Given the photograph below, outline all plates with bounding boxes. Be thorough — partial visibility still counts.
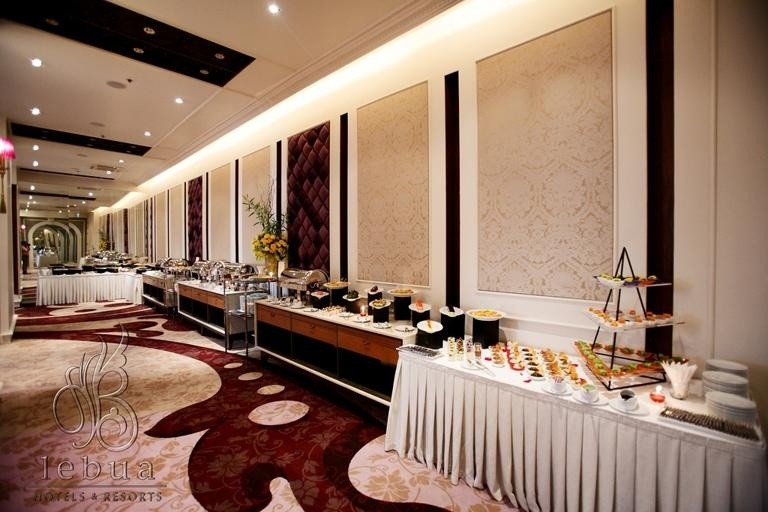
[271,299,317,313]
[238,293,267,314]
[489,339,648,416]
[144,271,176,281]
[183,278,232,291]
[703,391,757,424]
[311,280,506,337]
[703,359,748,374]
[700,371,750,396]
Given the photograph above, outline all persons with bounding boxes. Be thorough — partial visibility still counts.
[21,240,31,275]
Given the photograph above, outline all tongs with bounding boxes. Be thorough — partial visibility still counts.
[466,357,496,378]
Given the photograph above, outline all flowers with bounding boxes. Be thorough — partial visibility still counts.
[241,192,288,261]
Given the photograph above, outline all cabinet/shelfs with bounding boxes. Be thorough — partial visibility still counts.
[141,274,416,408]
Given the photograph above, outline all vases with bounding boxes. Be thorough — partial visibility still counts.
[265,256,278,278]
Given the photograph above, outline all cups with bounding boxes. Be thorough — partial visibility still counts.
[579,383,598,402]
[549,374,567,392]
[616,389,638,411]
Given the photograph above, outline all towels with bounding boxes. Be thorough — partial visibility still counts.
[660,359,698,396]
